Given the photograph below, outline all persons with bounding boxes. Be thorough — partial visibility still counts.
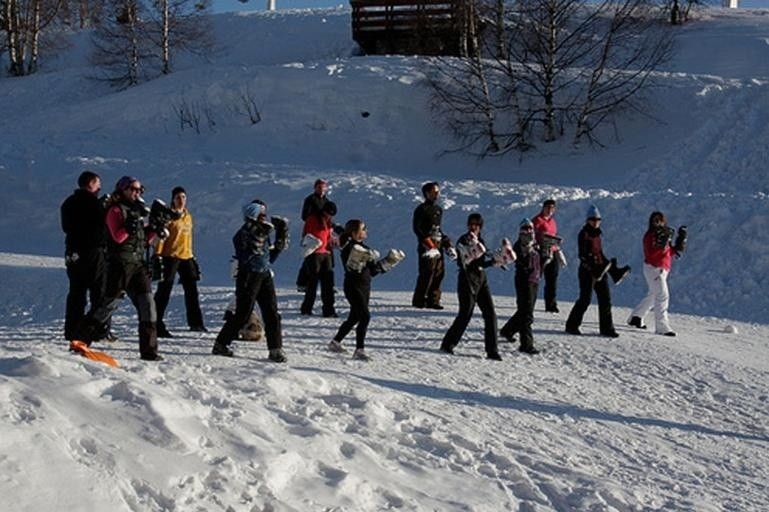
[297,179,342,292]
[627,211,687,337]
[532,199,567,314]
[60,171,117,341]
[301,202,342,318]
[565,205,632,338]
[225,200,272,341]
[413,182,458,309]
[441,213,517,360]
[71,171,166,362]
[328,220,405,362]
[152,187,208,337]
[500,217,542,353]
[212,200,290,363]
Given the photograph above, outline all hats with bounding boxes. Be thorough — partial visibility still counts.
[315,178,328,188]
[519,217,536,228]
[323,202,337,216]
[586,204,602,220]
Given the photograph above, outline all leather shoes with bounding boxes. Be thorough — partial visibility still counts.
[105,330,118,343]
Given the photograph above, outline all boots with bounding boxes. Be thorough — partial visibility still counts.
[213,341,234,356]
[301,234,322,257]
[421,238,441,260]
[126,195,150,238]
[608,258,632,287]
[329,233,351,250]
[519,231,536,258]
[444,240,457,263]
[492,238,518,267]
[378,248,406,273]
[150,256,165,283]
[677,225,689,252]
[335,224,344,234]
[654,224,674,247]
[457,231,487,265]
[541,232,563,256]
[585,258,612,282]
[180,261,201,284]
[268,349,286,363]
[245,214,274,247]
[346,245,380,273]
[270,215,290,251]
[148,198,182,240]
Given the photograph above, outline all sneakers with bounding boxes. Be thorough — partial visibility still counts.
[600,329,621,338]
[190,324,209,331]
[302,311,313,316]
[627,316,647,330]
[486,353,503,361]
[352,349,372,363]
[545,306,558,311]
[499,330,517,343]
[71,341,90,353]
[141,354,163,362]
[441,345,454,355]
[157,331,171,338]
[328,340,346,353]
[428,303,443,309]
[565,325,582,335]
[412,302,426,308]
[324,312,338,318]
[519,345,540,355]
[297,285,310,292]
[332,286,340,293]
[657,330,675,334]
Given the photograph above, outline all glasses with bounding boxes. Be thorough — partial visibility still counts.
[362,224,368,231]
[590,218,602,222]
[259,213,270,220]
[545,204,557,209]
[128,184,146,194]
